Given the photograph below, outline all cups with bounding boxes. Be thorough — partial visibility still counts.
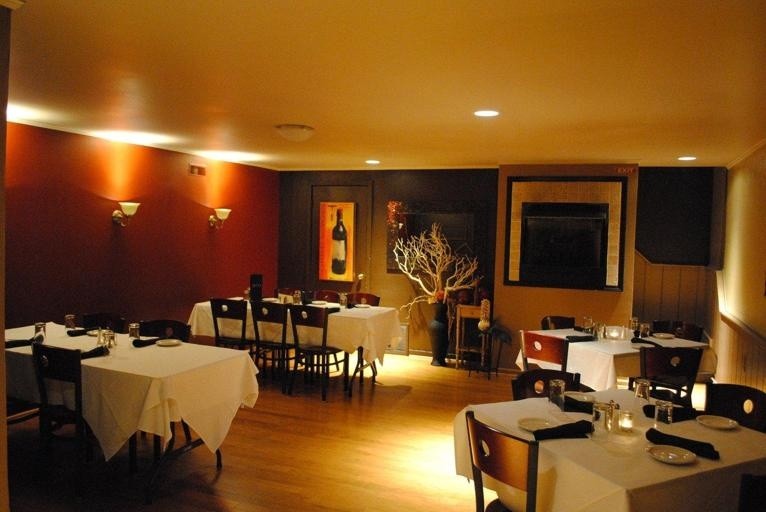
[634,379,650,408]
[654,400,673,431]
[596,322,606,340]
[65,315,76,333]
[583,317,594,333]
[628,316,639,330]
[639,324,650,339]
[35,322,46,342]
[128,323,141,344]
[591,402,614,442]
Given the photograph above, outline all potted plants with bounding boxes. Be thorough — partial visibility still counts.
[392,223,482,366]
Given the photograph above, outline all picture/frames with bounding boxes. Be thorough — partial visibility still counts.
[319,202,355,282]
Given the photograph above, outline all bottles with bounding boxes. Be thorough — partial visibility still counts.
[332,208,347,274]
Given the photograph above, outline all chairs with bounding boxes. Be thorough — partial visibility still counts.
[346,292,380,307]
[464,410,539,512]
[706,381,766,433]
[32,340,107,468]
[539,315,576,331]
[276,286,311,304]
[209,297,254,362]
[511,369,581,401]
[250,300,289,392]
[519,328,569,372]
[652,319,671,335]
[313,290,340,304]
[672,320,704,342]
[289,304,349,402]
[83,314,126,334]
[629,346,703,409]
[131,319,193,461]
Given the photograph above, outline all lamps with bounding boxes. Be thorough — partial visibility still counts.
[209,208,232,229]
[113,202,140,227]
[276,124,316,144]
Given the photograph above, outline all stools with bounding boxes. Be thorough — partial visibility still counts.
[455,302,491,369]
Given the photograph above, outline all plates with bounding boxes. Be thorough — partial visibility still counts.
[696,415,739,431]
[654,333,676,339]
[355,304,370,308]
[87,329,112,336]
[312,301,326,305]
[156,339,182,346]
[517,418,555,432]
[630,342,655,349]
[647,444,697,465]
[262,298,278,302]
[564,392,596,403]
